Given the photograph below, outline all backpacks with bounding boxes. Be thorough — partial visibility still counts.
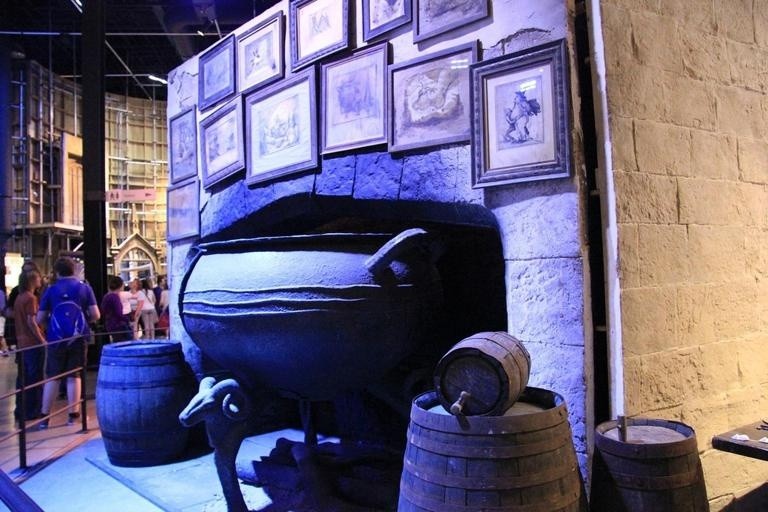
[47,281,90,360]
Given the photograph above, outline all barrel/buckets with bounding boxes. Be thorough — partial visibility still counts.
[94,337,195,468]
[394,385,589,512]
[433,330,532,419]
[587,411,711,512]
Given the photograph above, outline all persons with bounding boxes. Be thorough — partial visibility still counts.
[12,270,49,421]
[37,271,54,296]
[100,276,168,343]
[35,256,101,430]
[3,261,47,320]
[0,281,17,357]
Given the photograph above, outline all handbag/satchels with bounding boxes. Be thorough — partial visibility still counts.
[153,311,159,324]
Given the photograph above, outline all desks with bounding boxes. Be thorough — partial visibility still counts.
[710,417,768,463]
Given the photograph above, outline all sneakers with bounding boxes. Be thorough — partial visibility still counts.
[67,416,89,426]
[39,420,48,428]
[0,350,8,356]
